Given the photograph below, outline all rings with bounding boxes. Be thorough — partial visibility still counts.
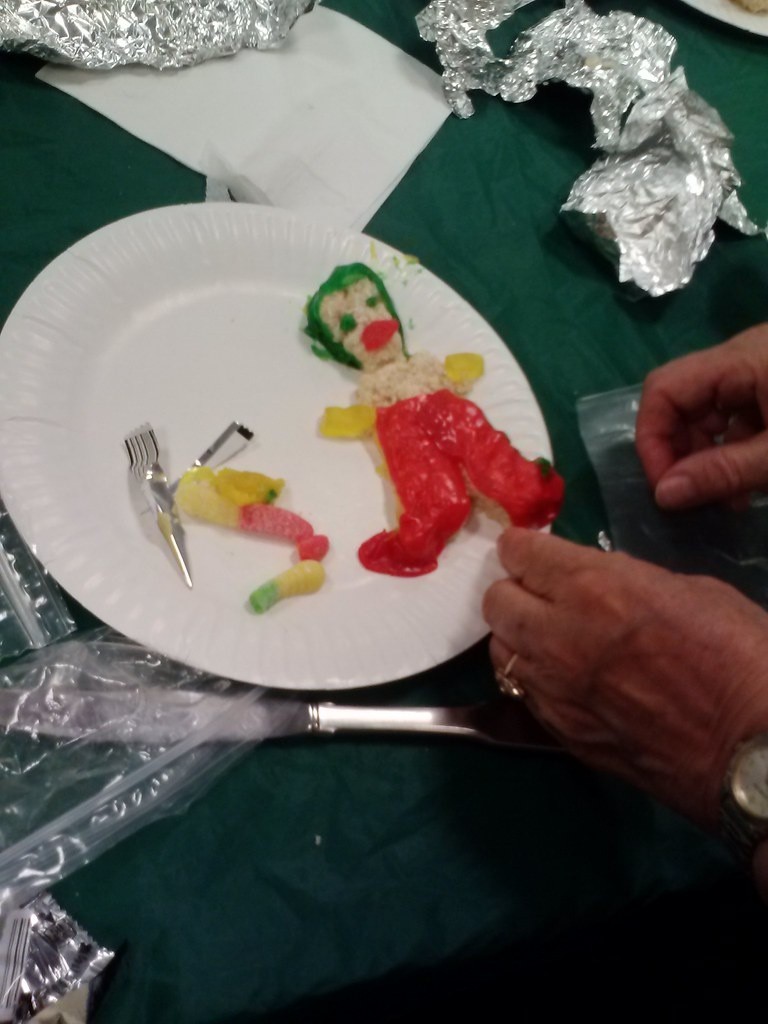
[495,653,524,698]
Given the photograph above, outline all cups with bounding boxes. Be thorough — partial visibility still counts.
[578,384,703,573]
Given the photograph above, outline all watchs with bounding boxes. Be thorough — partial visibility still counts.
[717,731,768,874]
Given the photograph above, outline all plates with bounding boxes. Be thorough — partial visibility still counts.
[681,0,768,48]
[1,201,559,692]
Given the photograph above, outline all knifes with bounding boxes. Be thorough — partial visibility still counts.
[0,683,553,753]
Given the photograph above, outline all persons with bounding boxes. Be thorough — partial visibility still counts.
[483,324,768,910]
